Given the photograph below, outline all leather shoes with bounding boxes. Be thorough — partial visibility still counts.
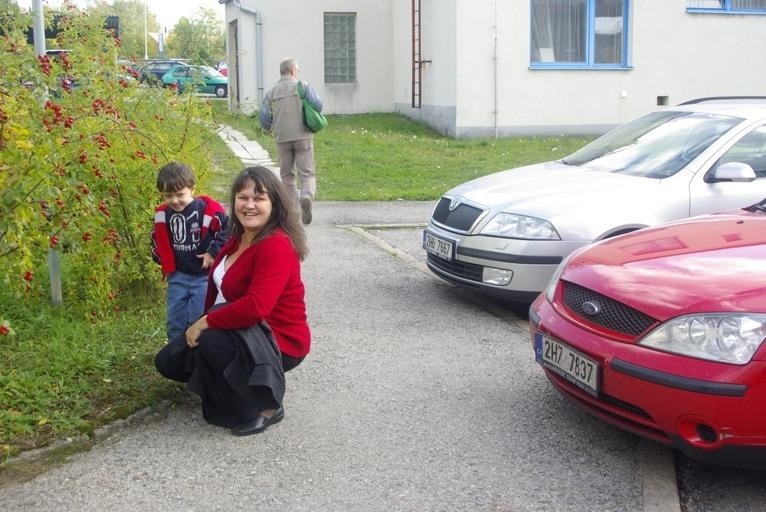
[231,405,285,436]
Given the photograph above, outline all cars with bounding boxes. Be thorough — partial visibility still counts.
[528,195,764,475]
[421,89,766,303]
[18,46,227,101]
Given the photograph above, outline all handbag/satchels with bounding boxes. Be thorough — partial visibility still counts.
[297,80,328,132]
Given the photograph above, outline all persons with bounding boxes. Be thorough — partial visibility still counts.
[151,162,228,347]
[154,167,310,435]
[260,58,323,225]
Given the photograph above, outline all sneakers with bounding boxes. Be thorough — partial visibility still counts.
[300,195,313,225]
